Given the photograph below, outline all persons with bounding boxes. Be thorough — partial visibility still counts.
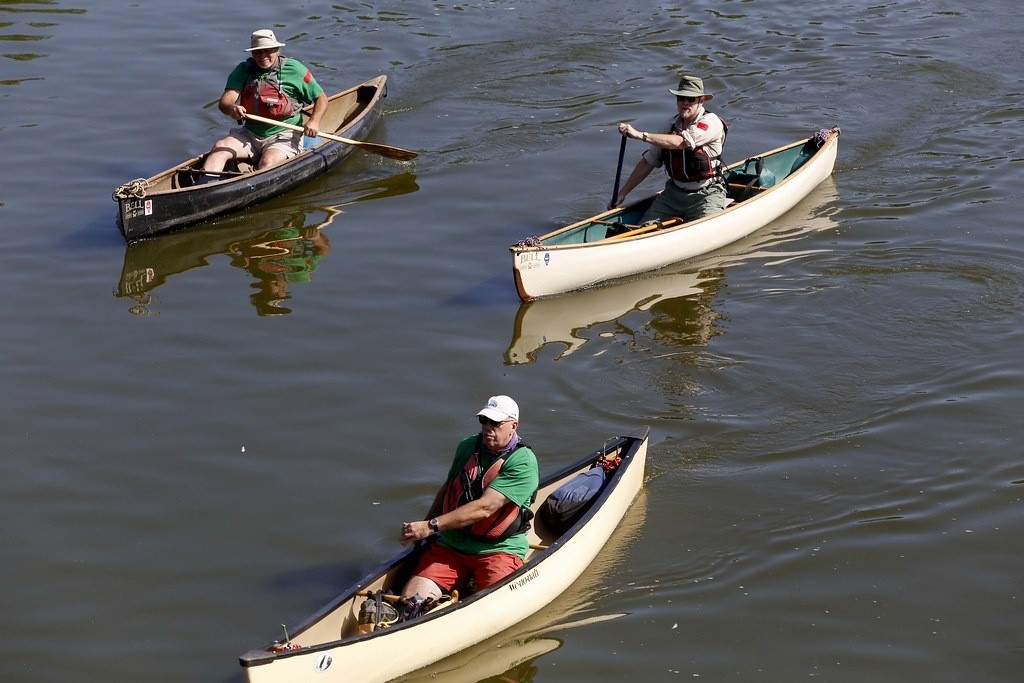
[197,28,329,186]
[606,75,728,234]
[399,396,540,607]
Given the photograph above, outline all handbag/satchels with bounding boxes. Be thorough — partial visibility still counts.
[726,157,775,202]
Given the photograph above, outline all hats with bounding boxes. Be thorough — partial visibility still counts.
[476,395,519,422]
[244,29,286,52]
[668,76,713,101]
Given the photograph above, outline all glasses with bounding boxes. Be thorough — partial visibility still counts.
[675,95,703,102]
[479,415,503,427]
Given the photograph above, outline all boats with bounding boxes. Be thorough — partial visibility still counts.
[508,123,840,299]
[118,127,382,299]
[237,422,650,683]
[111,72,388,241]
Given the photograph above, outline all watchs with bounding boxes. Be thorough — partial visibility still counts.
[429,518,439,533]
[643,132,649,142]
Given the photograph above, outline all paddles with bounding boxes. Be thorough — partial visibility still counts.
[243,113,419,161]
[610,134,627,209]
